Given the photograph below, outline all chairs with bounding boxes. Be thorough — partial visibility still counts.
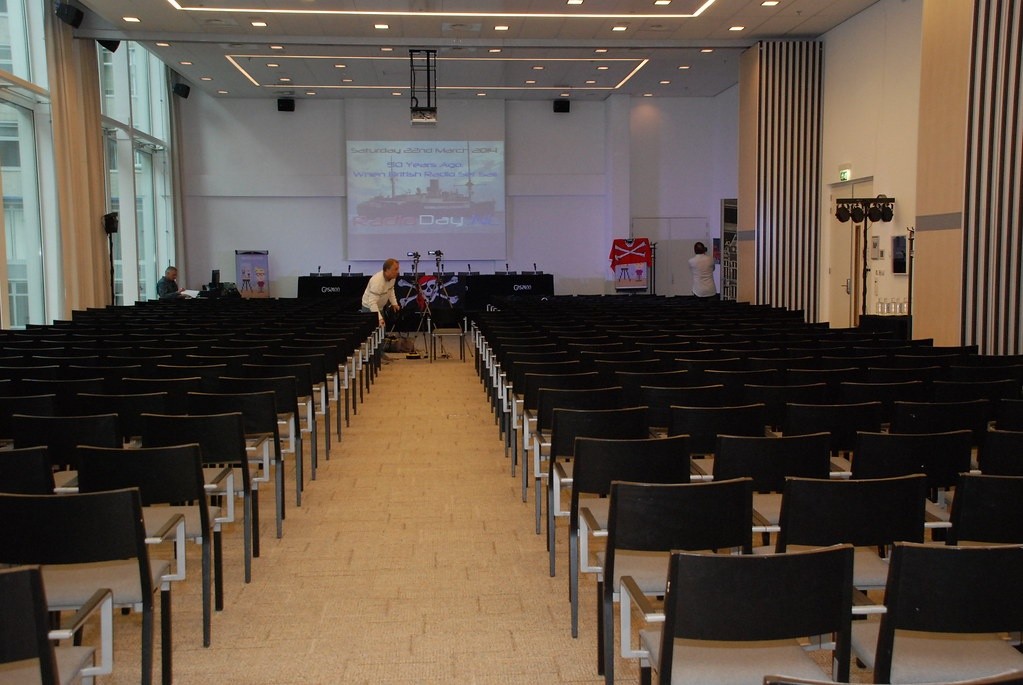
[0,294,1023,685]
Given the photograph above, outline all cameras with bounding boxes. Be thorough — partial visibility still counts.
[407,252,420,257]
[428,250,443,256]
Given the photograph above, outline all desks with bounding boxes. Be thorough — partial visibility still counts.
[297,277,555,325]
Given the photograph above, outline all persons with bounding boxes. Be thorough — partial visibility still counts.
[362,259,399,363]
[688,242,717,300]
[157,266,185,299]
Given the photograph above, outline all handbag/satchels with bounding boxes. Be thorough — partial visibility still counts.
[382,335,416,353]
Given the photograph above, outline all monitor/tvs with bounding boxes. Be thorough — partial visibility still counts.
[211,269,220,288]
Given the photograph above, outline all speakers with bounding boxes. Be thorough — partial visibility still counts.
[278,98,295,111]
[553,101,570,112]
[174,83,197,104]
[56,4,84,29]
[104,212,118,233]
[97,40,120,53]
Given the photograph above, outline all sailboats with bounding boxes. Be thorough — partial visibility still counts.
[357,141,494,222]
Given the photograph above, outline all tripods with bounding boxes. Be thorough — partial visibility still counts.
[382,256,474,358]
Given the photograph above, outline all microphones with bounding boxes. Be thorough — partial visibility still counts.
[318,266,322,270]
[412,264,414,272]
[506,264,508,269]
[467,264,470,270]
[442,264,444,268]
[534,263,536,270]
[348,265,351,270]
